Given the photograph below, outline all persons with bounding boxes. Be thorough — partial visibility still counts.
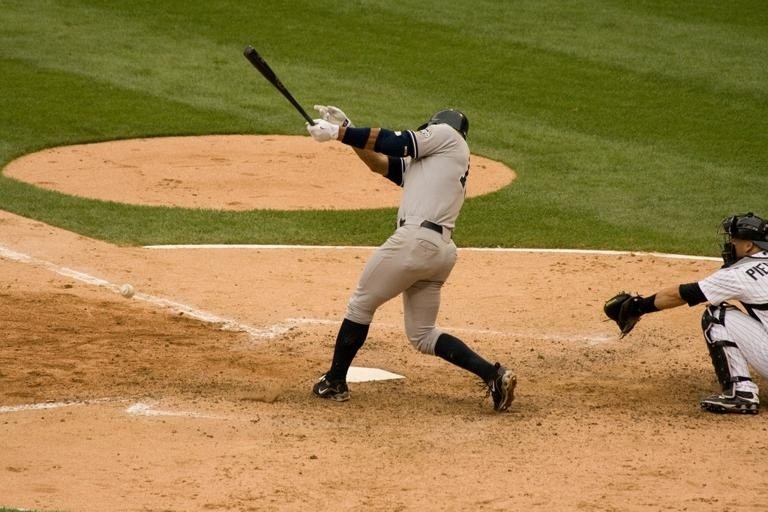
[603,210,767,417]
[306,103,520,414]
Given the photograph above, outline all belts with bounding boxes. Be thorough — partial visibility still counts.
[396,218,452,237]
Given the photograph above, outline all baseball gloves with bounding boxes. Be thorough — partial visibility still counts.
[604,292,644,338]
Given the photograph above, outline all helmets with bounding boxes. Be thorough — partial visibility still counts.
[416,107,468,139]
[715,213,768,268]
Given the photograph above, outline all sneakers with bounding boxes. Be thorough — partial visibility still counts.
[700,395,759,414]
[487,362,516,411]
[314,379,350,401]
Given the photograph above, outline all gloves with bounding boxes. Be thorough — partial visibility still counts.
[313,103,355,128]
[305,120,339,142]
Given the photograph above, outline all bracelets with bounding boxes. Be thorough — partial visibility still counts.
[342,124,371,151]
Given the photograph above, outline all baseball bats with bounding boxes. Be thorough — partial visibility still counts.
[244,45,314,125]
